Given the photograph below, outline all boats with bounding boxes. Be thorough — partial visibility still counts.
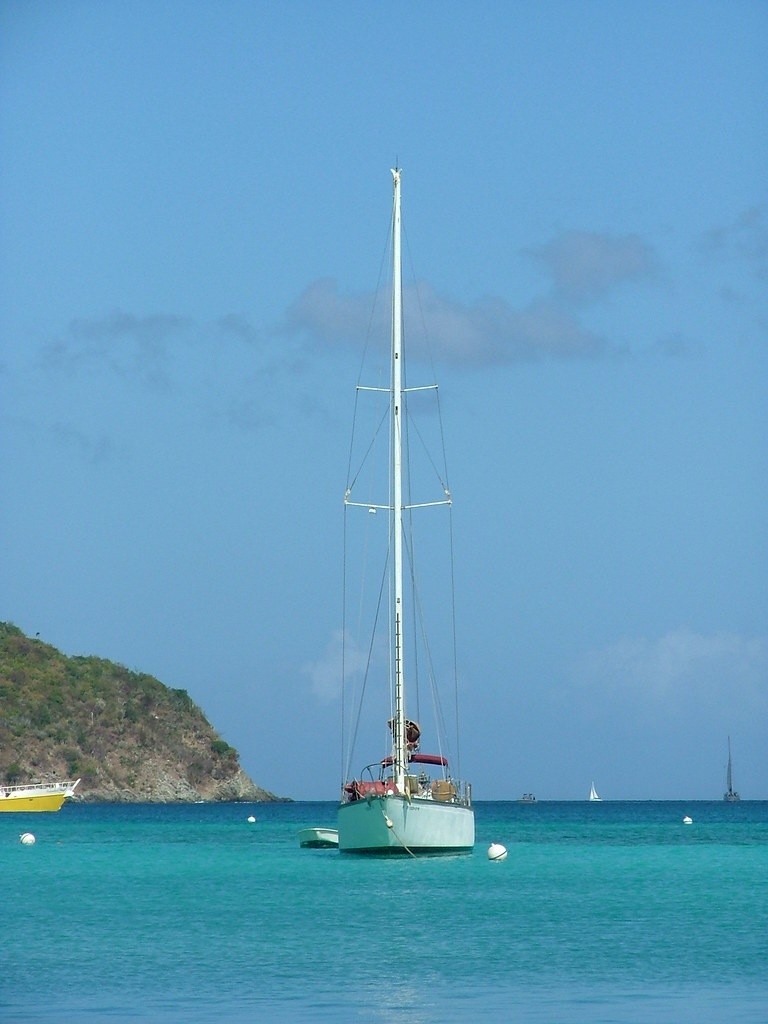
[517,791,538,804]
[0,778,82,813]
[297,828,339,845]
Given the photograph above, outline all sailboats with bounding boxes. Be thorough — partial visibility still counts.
[588,781,603,801]
[723,735,740,802]
[334,161,476,851]
